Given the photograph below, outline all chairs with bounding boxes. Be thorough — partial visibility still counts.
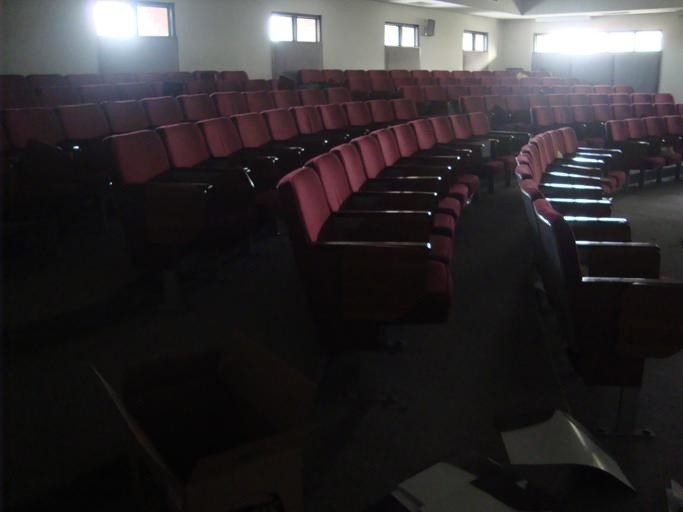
[0,68,683,368]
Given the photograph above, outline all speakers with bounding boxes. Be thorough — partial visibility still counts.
[425,19,435,36]
